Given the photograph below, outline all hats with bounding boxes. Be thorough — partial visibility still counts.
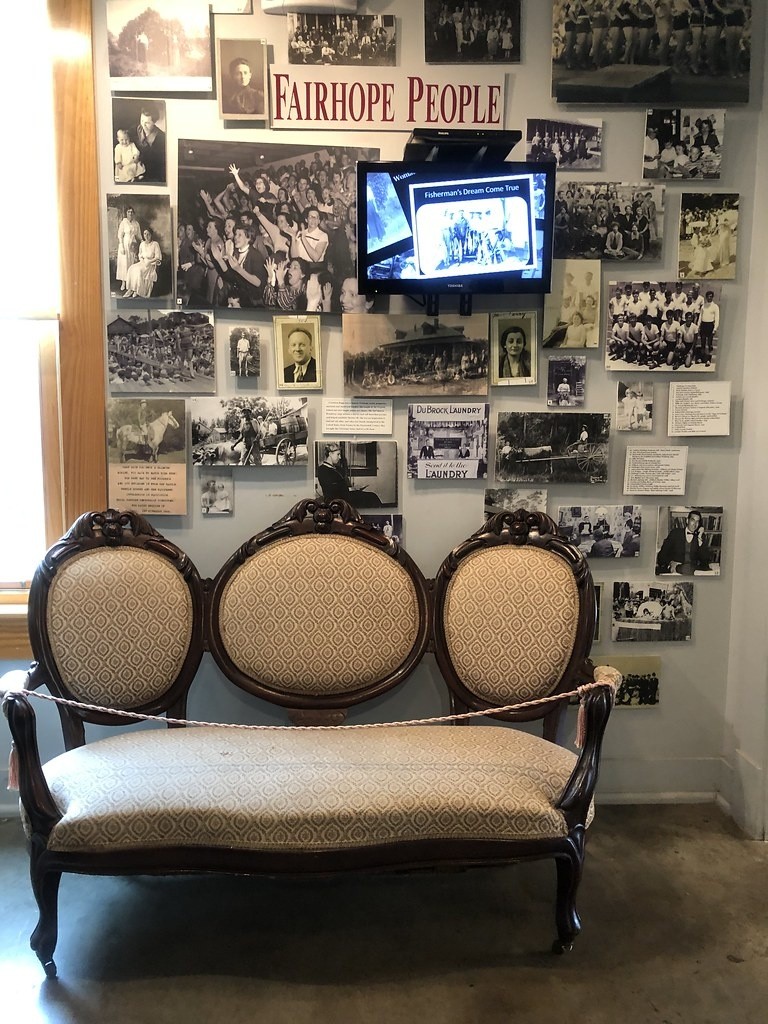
[140,399,148,406]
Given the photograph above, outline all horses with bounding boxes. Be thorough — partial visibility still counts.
[116,410,180,463]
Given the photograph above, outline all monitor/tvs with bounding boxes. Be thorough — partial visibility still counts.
[354,161,556,294]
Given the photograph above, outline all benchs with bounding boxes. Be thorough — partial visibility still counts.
[0,499,622,976]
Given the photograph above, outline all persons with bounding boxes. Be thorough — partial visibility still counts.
[419,439,434,459]
[317,444,381,506]
[615,672,659,705]
[557,378,570,405]
[458,444,470,459]
[137,399,154,445]
[579,512,640,557]
[372,520,393,539]
[231,408,277,466]
[202,480,232,512]
[622,387,646,429]
[657,509,709,575]
[613,592,681,621]
[571,424,588,453]
[95,0,753,390]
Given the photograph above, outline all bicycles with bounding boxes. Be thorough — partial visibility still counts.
[362,367,393,389]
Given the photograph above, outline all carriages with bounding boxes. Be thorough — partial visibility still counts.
[191,426,307,467]
[501,440,610,475]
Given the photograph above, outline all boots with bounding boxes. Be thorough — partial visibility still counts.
[144,435,150,446]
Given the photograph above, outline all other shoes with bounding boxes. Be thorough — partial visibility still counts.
[608,353,712,369]
[120,283,125,290]
[123,290,132,297]
[133,292,137,297]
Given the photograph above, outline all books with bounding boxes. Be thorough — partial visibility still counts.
[670,515,721,562]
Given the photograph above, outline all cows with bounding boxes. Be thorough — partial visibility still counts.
[515,445,552,476]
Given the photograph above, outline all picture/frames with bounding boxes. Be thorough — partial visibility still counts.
[217,37,269,121]
[491,311,537,386]
[273,315,323,390]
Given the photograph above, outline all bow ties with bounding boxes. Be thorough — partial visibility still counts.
[687,532,695,535]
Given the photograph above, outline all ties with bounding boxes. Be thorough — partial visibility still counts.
[296,366,304,382]
